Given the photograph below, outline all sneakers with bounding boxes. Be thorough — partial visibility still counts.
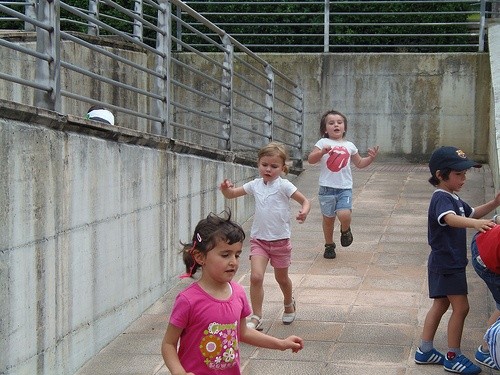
[414,346,446,365]
[474,344,499,370]
[443,352,482,375]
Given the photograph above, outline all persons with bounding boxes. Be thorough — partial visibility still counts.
[308,110,379,259]
[162,207,304,375]
[219,142,311,331]
[414,145,500,373]
[470,214,500,372]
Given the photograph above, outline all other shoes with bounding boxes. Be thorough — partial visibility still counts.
[246,314,264,332]
[282,297,296,323]
[340,225,353,247]
[323,242,336,259]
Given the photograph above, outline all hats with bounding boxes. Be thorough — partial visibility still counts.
[429,145,483,176]
